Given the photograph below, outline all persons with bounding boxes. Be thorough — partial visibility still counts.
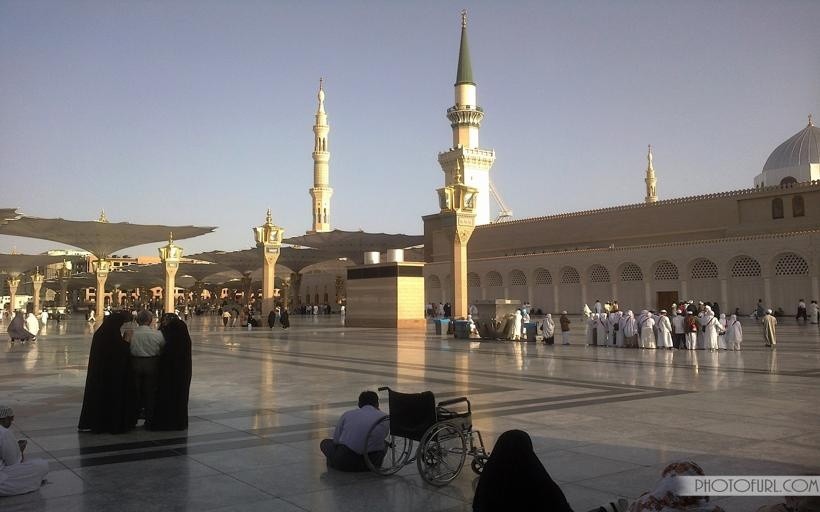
[425,299,572,346]
[587,348,780,393]
[0,304,62,343]
[75,300,346,331]
[0,405,50,497]
[319,389,390,473]
[76,311,194,434]
[581,296,820,352]
[470,428,820,512]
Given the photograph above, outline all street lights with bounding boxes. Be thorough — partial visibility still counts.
[6,209,285,327]
[436,158,479,320]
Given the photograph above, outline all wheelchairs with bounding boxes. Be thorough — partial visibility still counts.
[364,386,491,487]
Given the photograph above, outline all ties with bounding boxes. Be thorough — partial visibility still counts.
[0,406,15,419]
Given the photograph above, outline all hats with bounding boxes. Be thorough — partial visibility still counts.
[672,303,677,308]
[766,309,772,314]
[677,310,682,313]
[660,309,667,313]
[562,311,568,315]
[687,300,694,304]
[686,311,693,315]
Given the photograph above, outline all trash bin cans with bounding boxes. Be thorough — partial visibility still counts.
[526,323,537,342]
[434,319,449,335]
[454,320,469,339]
[448,321,453,334]
[466,322,472,336]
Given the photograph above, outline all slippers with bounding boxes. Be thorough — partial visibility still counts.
[586,498,628,512]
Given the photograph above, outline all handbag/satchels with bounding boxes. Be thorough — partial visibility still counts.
[702,325,705,332]
[638,329,641,334]
[719,332,725,335]
[540,325,543,330]
[614,323,619,331]
